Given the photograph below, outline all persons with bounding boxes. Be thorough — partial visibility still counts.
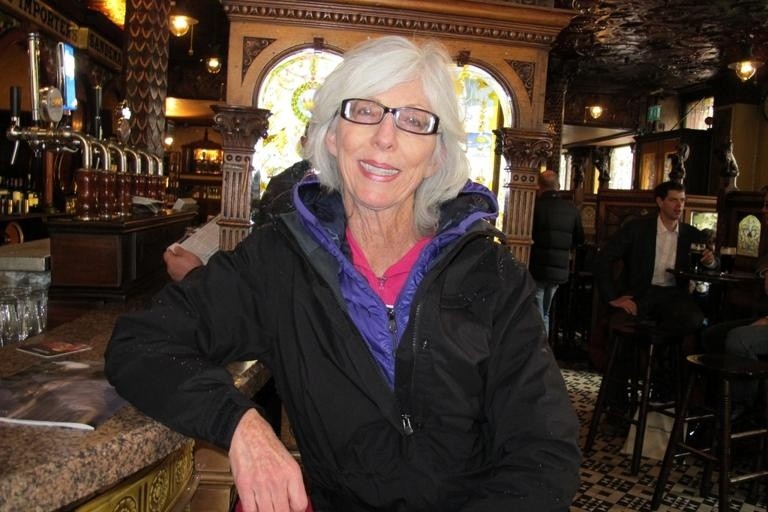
[102,33,583,512]
[595,181,721,424]
[530,168,583,340]
[161,119,311,285]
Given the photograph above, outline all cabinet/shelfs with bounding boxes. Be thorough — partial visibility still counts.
[626,125,711,192]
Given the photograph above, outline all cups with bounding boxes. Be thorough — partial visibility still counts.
[719,247,737,273]
[1,284,47,345]
[688,243,707,273]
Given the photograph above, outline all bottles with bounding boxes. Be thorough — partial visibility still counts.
[251,171,260,199]
[194,150,222,176]
[195,185,222,209]
[1,170,38,215]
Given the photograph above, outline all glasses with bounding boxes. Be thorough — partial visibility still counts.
[332,95,441,137]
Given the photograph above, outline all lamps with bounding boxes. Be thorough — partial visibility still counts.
[723,33,765,85]
[163,0,198,38]
[577,87,608,121]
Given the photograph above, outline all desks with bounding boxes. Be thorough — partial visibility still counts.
[665,264,766,306]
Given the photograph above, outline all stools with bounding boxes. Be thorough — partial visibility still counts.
[583,317,686,474]
[653,347,766,511]
